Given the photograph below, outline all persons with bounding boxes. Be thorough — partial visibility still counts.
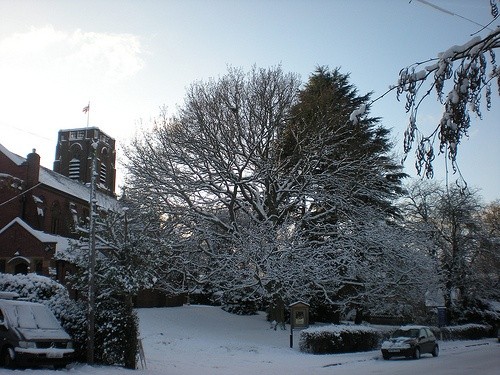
[269,319,287,332]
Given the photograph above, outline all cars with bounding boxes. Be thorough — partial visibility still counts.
[381,324,439,359]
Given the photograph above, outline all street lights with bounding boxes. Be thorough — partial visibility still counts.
[66,138,111,362]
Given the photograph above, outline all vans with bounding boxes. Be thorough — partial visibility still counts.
[0,290,73,370]
